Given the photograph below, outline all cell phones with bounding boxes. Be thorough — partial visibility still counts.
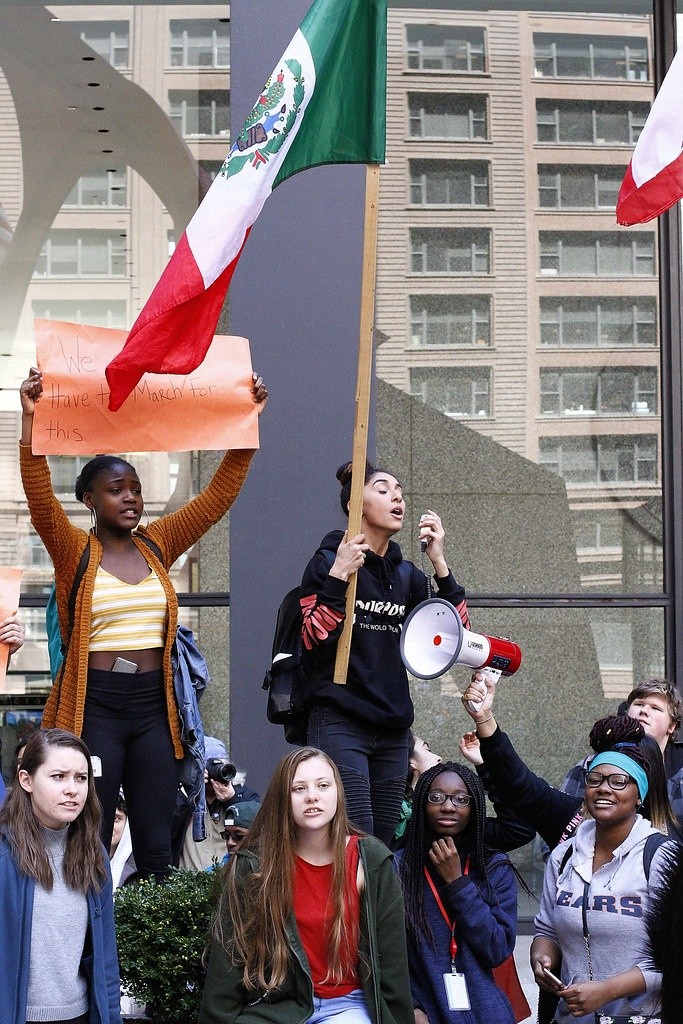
[111,657,138,674]
[543,968,564,988]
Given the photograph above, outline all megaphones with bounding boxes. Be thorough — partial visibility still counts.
[400,598,522,713]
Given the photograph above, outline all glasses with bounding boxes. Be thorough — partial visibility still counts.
[424,792,472,808]
[17,758,22,763]
[584,771,635,791]
[220,831,246,841]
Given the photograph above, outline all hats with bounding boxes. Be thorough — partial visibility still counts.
[204,737,231,762]
[221,800,261,828]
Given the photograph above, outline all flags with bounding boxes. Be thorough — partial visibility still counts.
[616,45,682,226]
[105,0,387,412]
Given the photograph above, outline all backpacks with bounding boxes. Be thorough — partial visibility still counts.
[46,536,162,684]
[263,550,412,724]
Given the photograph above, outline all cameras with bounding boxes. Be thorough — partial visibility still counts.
[203,757,236,793]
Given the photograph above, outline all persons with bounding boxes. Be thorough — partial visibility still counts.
[1,728,138,1024]
[391,763,517,1024]
[462,673,682,852]
[393,729,538,854]
[177,737,263,874]
[20,364,270,885]
[0,610,23,673]
[201,747,416,1024]
[299,462,470,853]
[529,679,683,1024]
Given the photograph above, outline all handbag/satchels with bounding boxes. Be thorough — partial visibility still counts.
[594,1012,666,1024]
[489,959,533,1023]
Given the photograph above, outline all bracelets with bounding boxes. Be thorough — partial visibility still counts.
[476,716,493,724]
